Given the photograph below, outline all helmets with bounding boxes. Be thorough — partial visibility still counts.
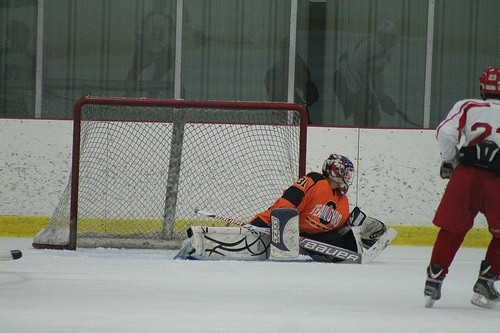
[479,67,500,94]
[322,154,354,193]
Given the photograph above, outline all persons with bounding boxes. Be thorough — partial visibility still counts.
[331,19,399,126]
[122,0,204,99]
[4,16,55,116]
[424,67,500,309]
[261,32,321,125]
[247,155,385,263]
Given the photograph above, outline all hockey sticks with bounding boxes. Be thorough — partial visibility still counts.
[192,207,399,263]
[0,249,24,262]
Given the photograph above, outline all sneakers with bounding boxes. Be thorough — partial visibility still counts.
[424,266,446,309]
[471,260,500,309]
